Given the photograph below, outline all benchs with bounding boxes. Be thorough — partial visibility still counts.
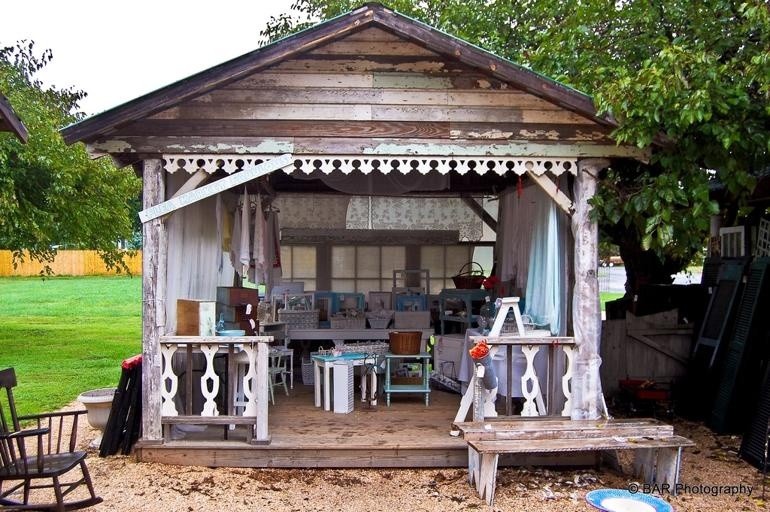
[454,415,687,506]
[159,413,257,445]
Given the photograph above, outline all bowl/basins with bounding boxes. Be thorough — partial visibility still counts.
[218,330,246,336]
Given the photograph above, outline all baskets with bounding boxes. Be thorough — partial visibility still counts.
[391,378,423,385]
[277,293,320,330]
[451,261,486,289]
[346,342,390,375]
[301,356,315,385]
[489,314,535,333]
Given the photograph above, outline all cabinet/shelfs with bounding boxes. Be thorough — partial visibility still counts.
[383,351,432,408]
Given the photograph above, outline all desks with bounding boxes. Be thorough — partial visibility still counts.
[309,352,380,412]
[283,326,435,384]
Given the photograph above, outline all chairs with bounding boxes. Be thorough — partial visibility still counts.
[0,366,104,512]
[261,320,295,406]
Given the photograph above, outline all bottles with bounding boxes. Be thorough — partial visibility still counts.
[479,295,498,335]
[217,312,225,330]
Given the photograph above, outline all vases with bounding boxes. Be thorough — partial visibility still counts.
[77,388,117,448]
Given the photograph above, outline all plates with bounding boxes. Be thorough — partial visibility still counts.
[585,488,673,512]
